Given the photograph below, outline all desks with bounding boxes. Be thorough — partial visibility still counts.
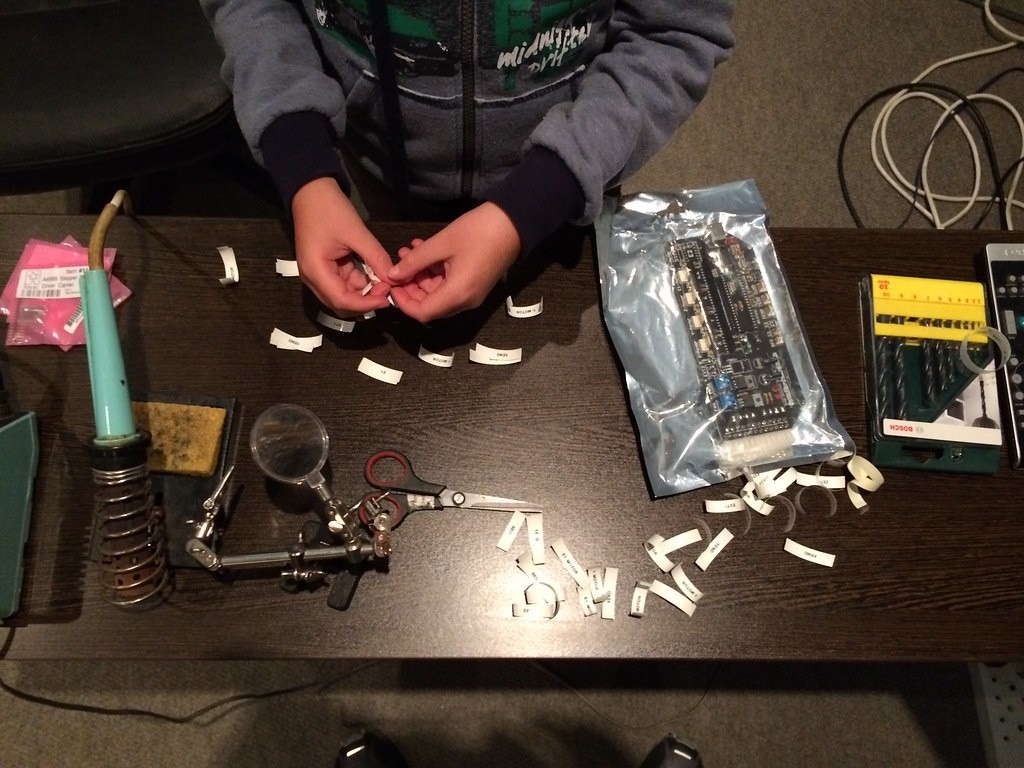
[1,213,1024,665]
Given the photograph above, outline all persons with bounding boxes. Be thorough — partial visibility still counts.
[213,0,734,324]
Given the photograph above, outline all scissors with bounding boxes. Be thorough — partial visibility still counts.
[360,449,544,532]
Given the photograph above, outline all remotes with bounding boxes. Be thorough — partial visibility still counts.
[985,241,1024,470]
